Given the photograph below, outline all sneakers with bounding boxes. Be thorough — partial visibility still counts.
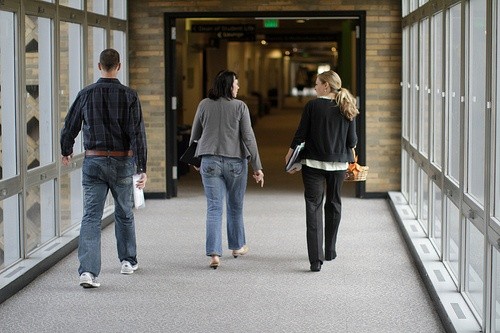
[79,272,101,288]
[120,260,139,275]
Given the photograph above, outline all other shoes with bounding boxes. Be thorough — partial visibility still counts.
[310,261,321,271]
[324,256,336,261]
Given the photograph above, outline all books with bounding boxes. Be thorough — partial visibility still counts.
[285,145,304,171]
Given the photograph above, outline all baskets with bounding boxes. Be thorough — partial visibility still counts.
[343,149,370,183]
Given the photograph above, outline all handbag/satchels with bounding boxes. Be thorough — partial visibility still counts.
[179,139,203,168]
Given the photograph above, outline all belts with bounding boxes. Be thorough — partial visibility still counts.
[85,149,133,157]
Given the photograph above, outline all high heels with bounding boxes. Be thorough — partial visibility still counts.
[232,244,249,259]
[209,263,220,270]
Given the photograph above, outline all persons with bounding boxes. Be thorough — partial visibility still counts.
[189,70,264,269]
[60,48,148,288]
[295,65,308,101]
[286,71,359,272]
